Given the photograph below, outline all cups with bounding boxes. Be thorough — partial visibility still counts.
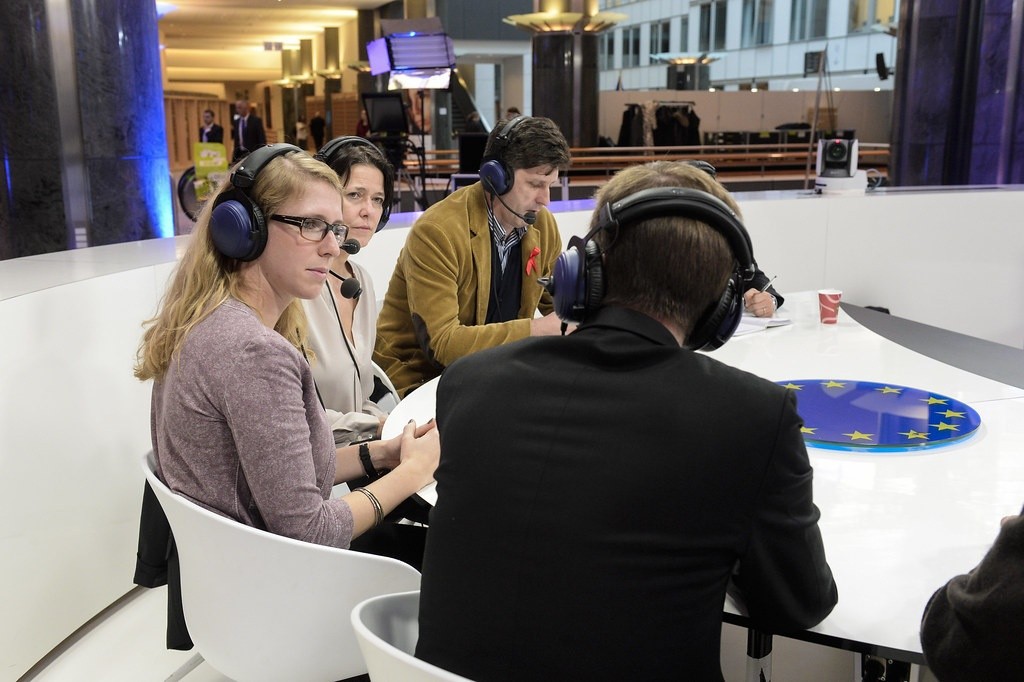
[819,289,842,324]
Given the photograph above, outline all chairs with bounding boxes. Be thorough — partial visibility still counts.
[349,591,472,682]
[140,449,421,682]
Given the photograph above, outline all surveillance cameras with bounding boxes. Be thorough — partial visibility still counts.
[814,136,869,196]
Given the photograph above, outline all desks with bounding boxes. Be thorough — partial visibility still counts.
[395,154,891,211]
[548,168,889,202]
[380,290,1024,682]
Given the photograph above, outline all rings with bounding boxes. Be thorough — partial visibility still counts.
[763,308,766,315]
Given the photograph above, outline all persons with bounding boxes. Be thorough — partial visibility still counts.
[464,111,483,132]
[199,109,224,144]
[231,99,267,163]
[413,160,839,682]
[370,114,578,398]
[684,161,785,318]
[507,107,521,115]
[356,110,369,137]
[298,137,389,444]
[133,141,440,682]
[296,111,326,154]
[920,505,1024,682]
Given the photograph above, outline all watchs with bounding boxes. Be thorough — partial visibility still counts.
[771,294,777,311]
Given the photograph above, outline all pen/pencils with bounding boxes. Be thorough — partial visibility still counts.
[759,275,777,293]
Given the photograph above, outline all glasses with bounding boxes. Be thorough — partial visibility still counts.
[265,212,351,246]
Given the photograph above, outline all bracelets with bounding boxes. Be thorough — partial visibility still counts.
[353,488,384,531]
[359,442,378,477]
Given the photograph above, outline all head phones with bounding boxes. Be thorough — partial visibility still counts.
[536,186,756,350]
[478,115,534,196]
[208,142,307,263]
[313,135,391,234]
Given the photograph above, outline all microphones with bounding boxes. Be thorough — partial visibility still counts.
[485,176,536,224]
[339,239,361,254]
[329,271,362,300]
